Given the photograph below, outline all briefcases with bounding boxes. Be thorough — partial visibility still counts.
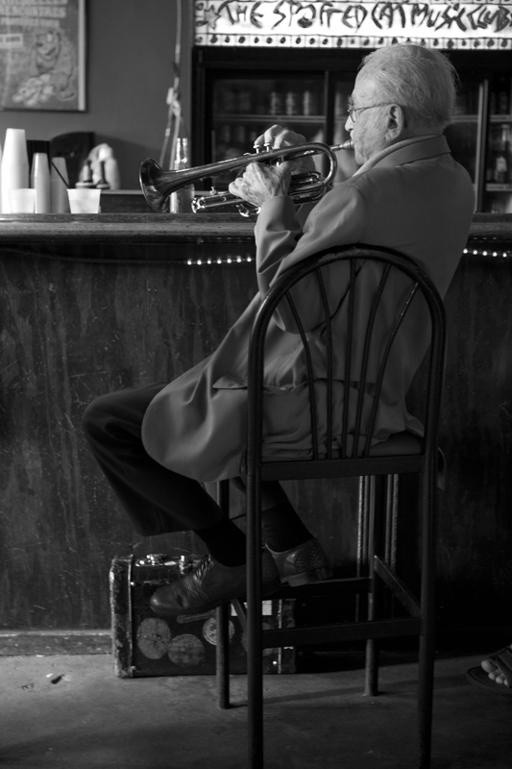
[109,555,298,678]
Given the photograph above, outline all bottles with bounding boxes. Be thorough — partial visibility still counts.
[493,124,511,183]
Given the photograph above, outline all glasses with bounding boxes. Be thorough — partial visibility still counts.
[345,101,408,122]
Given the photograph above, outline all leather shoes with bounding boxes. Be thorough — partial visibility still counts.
[150,552,261,616]
[264,540,332,584]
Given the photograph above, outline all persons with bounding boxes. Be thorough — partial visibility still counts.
[82,44,475,617]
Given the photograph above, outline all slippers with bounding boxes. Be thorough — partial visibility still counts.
[466,648,512,693]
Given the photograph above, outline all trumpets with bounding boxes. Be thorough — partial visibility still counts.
[139,135,354,213]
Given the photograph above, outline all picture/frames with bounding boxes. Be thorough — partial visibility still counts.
[0,0,88,114]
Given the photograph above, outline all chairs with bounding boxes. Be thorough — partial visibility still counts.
[216,240,447,769]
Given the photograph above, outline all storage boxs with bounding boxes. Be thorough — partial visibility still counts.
[107,553,247,676]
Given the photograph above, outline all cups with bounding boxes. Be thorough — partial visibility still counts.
[1,126,101,215]
